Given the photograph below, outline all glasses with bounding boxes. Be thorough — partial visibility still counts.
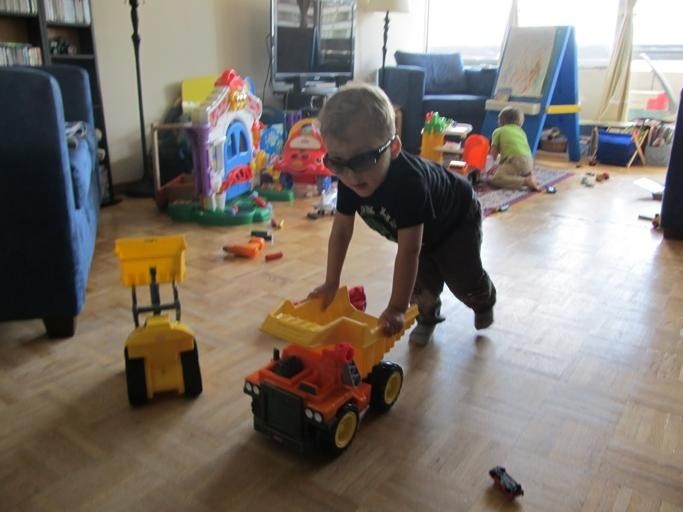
[320,141,393,172]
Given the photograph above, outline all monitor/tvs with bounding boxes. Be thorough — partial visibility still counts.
[270,0,356,80]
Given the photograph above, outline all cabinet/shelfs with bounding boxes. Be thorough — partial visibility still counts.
[273,88,338,125]
[0,0,115,204]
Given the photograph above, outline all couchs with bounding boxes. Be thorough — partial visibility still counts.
[375,50,499,145]
[1,59,101,342]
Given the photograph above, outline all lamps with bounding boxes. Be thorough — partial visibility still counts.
[366,0,412,89]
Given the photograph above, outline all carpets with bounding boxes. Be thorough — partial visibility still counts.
[468,150,574,223]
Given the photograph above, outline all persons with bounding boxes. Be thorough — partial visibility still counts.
[305,80,497,349]
[487,106,538,192]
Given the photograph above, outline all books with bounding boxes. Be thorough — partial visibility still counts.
[0,0,92,67]
